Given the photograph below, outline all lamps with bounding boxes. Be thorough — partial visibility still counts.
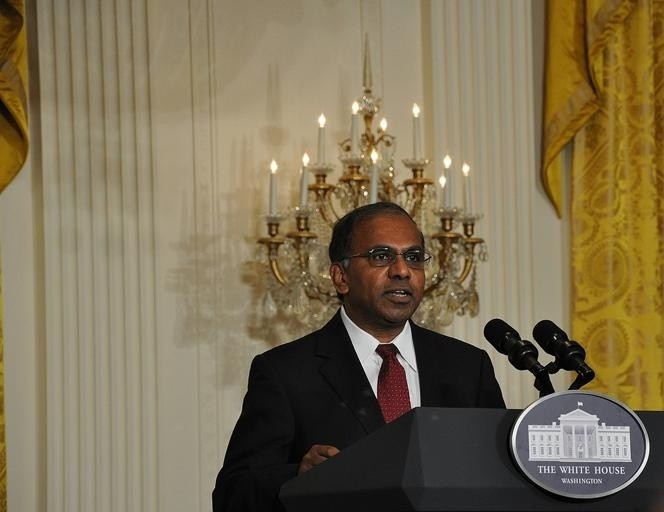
[260,32,485,333]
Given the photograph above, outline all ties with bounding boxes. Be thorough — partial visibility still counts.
[374,344,412,425]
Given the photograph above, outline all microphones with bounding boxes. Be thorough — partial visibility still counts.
[535,315,591,371]
[486,318,545,369]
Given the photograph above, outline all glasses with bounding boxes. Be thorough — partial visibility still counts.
[338,247,433,270]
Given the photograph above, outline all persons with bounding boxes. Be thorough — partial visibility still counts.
[209,199,509,511]
